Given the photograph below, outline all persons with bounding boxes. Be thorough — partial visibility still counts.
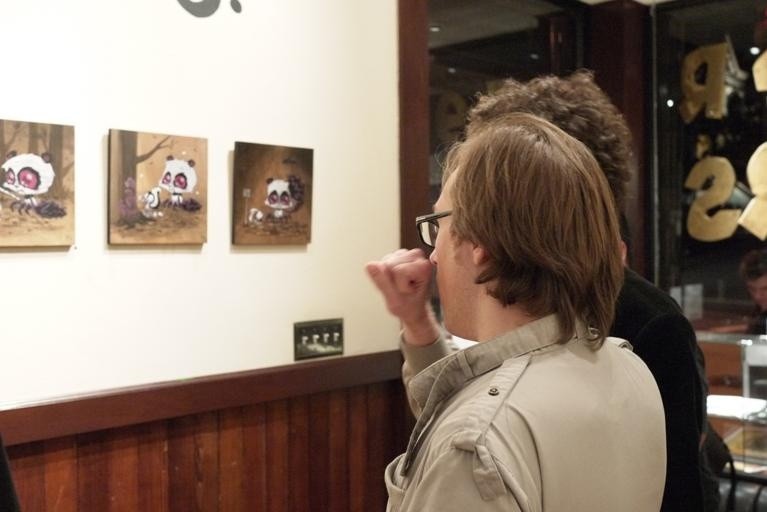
[463,72,722,510]
[361,108,668,511]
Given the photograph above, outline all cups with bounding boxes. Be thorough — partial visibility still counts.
[416,208,454,247]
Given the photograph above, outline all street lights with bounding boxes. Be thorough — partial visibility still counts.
[701,428,736,512]
